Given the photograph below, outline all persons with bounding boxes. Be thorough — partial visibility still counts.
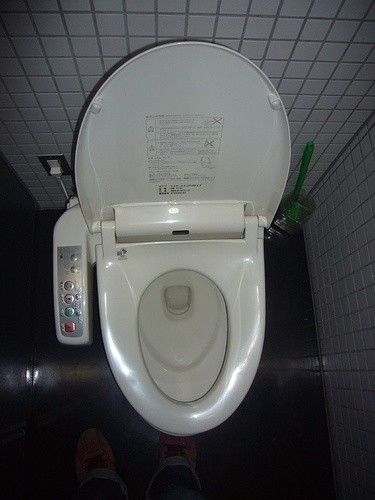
[73,426,205,500]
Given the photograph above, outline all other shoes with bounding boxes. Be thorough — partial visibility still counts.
[155,430,197,467]
[73,426,127,498]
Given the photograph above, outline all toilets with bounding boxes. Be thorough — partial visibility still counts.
[51,40,296,440]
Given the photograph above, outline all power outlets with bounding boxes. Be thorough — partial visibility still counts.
[47,160,64,174]
